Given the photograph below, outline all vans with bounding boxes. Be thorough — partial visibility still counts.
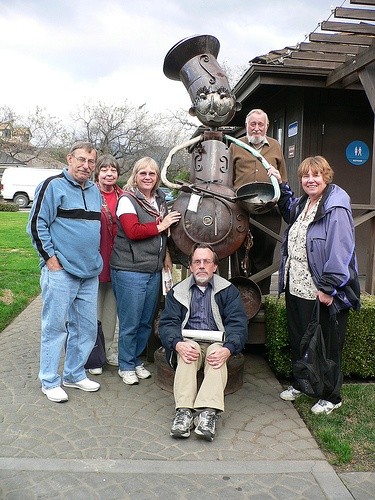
[0,166,63,208]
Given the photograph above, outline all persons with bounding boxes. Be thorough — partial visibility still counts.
[27,142,181,403]
[230,109,360,415]
[159,242,248,441]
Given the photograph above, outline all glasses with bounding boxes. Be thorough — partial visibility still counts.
[71,153,98,168]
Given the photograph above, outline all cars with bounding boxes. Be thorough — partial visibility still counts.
[158,187,174,201]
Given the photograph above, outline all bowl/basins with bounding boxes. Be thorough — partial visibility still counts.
[235,181,278,216]
[227,277,261,320]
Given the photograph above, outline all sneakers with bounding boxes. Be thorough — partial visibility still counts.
[136,365,154,380]
[63,375,104,391]
[281,388,302,402]
[170,411,194,440]
[41,383,68,403]
[117,368,137,385]
[86,367,106,373]
[102,349,118,367]
[193,409,215,439]
[310,400,346,415]
